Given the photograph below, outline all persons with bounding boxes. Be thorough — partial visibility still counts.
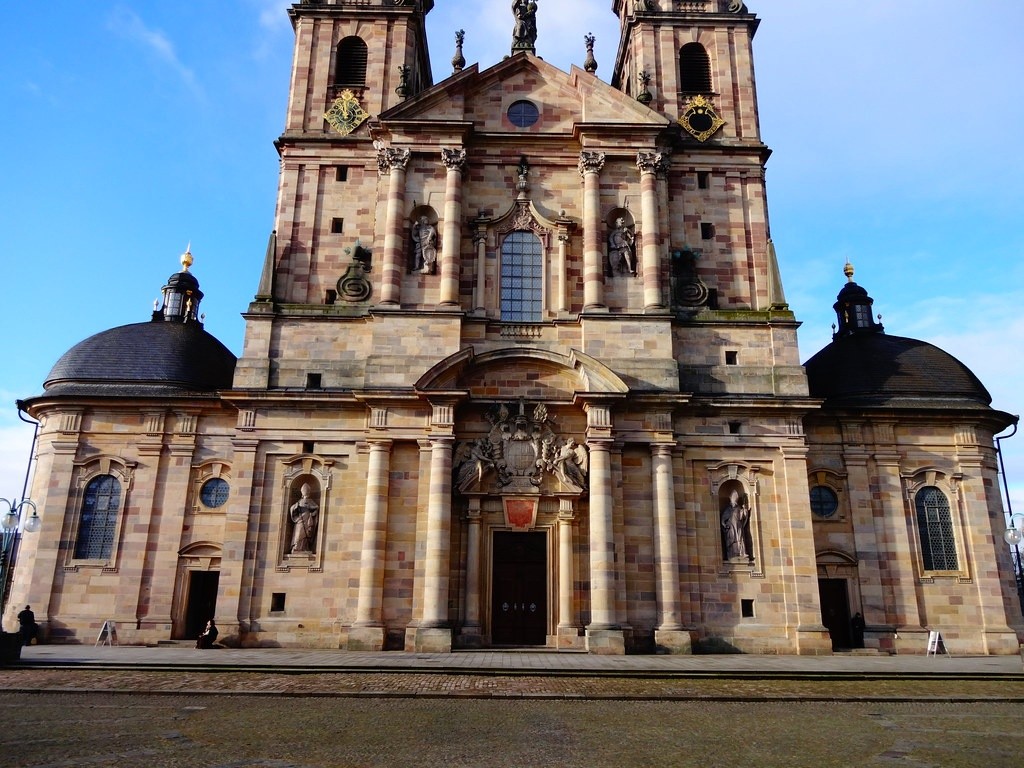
[471,438,493,482]
[289,483,319,551]
[609,217,635,276]
[412,215,437,275]
[512,0,537,40]
[554,437,584,484]
[194,620,219,649]
[721,490,749,559]
[851,612,865,649]
[18,605,35,632]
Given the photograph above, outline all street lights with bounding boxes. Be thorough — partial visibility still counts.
[0,498,42,632]
